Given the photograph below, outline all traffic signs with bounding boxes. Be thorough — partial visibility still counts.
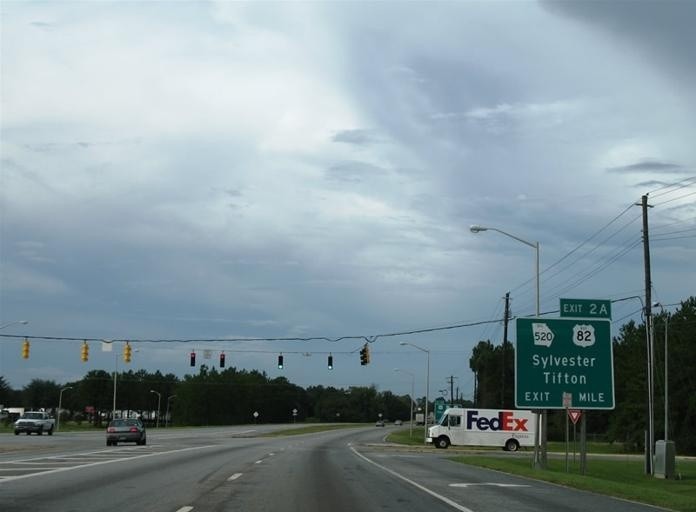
[514,316,616,411]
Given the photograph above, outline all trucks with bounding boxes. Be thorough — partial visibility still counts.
[415,412,425,426]
[425,407,542,452]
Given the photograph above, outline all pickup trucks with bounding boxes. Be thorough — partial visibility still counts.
[13,411,56,436]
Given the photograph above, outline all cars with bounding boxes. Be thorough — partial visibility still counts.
[383,418,389,423]
[105,418,146,446]
[394,419,403,425]
[376,420,384,427]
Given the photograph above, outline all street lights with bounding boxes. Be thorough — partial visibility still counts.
[653,302,671,440]
[150,390,161,427]
[470,223,543,460]
[0,320,28,329]
[165,395,176,428]
[392,368,413,438]
[112,349,141,419]
[399,342,429,442]
[56,386,72,432]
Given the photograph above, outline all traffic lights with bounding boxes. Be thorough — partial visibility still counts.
[360,346,370,365]
[278,355,283,369]
[219,354,225,367]
[124,345,131,362]
[80,344,89,362]
[327,355,334,369]
[190,353,195,366]
